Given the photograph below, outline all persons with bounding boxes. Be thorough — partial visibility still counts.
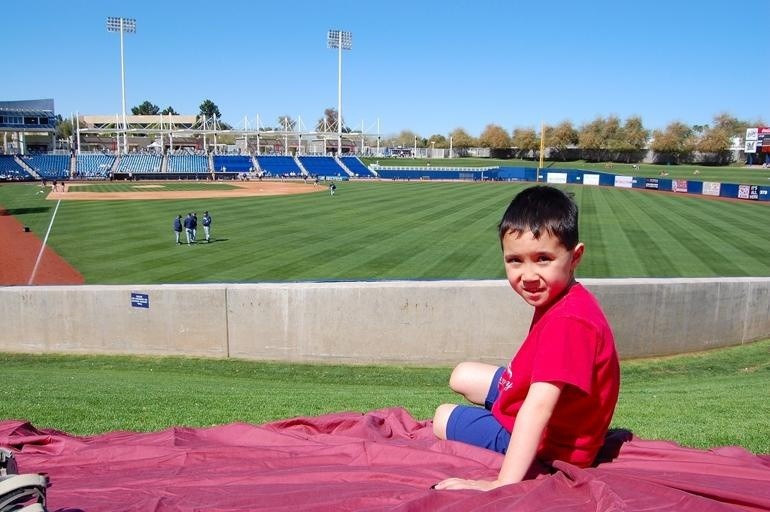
[220,164,227,172]
[0,169,24,182]
[238,168,340,199]
[41,168,69,192]
[432,185,621,493]
[173,214,183,245]
[200,210,212,243]
[72,164,134,181]
[183,213,197,247]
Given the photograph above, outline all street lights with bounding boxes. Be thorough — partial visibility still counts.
[325,29,352,158]
[105,16,136,157]
